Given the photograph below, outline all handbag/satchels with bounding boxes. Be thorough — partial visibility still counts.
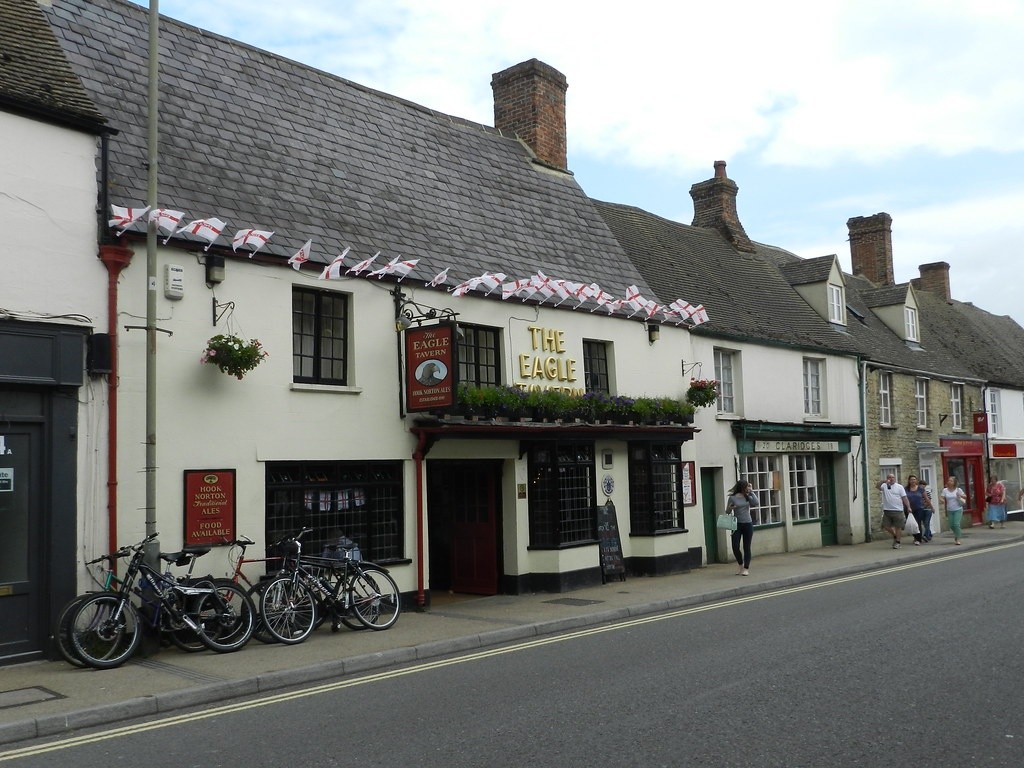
[986,497,991,503]
[717,509,737,535]
[956,492,967,506]
[903,513,920,535]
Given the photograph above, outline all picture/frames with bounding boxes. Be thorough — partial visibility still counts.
[183,469,237,548]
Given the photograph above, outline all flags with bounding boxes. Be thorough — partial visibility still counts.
[291,241,311,270]
[367,256,419,276]
[346,252,381,275]
[177,218,225,242]
[319,249,349,279]
[109,204,148,227]
[432,267,710,326]
[149,209,183,231]
[232,229,273,252]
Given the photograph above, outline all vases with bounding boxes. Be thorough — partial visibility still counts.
[431,404,694,426]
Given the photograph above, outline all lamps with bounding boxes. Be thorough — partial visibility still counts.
[648,324,660,340]
[205,254,225,282]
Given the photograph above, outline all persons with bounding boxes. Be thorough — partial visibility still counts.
[918,480,932,541]
[726,481,759,575]
[876,474,912,549]
[941,475,966,545]
[985,475,1006,528]
[904,475,929,546]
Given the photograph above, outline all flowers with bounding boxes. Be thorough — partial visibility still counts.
[449,383,694,420]
[685,377,719,408]
[200,335,269,380]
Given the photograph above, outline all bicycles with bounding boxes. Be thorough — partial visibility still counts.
[47,530,408,671]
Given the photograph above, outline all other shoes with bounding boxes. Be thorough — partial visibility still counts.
[1000,523,1006,528]
[743,571,749,576]
[989,521,995,529]
[923,536,929,542]
[955,537,961,544]
[735,568,741,575]
[891,538,901,549]
[913,537,921,545]
[929,534,933,540]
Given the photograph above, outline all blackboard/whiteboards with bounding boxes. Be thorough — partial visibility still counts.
[597,504,627,575]
